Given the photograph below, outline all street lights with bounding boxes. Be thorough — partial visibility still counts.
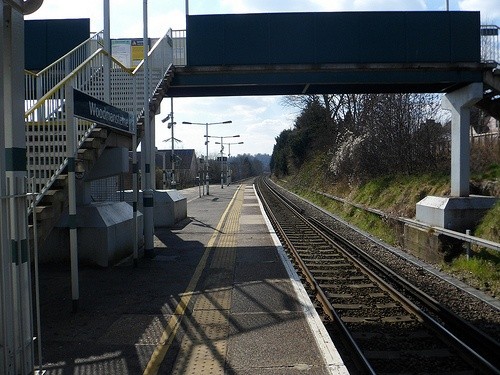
[204,134,240,189]
[215,142,244,188]
[181,120,233,196]
